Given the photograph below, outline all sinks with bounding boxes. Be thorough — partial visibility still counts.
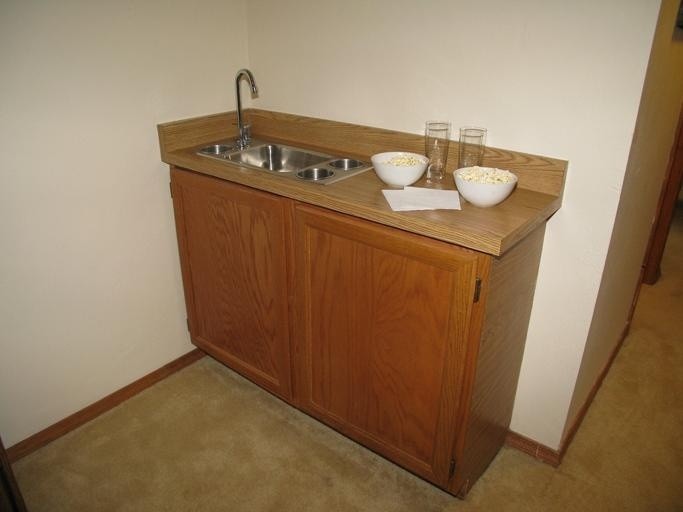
[198,134,373,187]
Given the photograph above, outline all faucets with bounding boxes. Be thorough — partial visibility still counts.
[235,69,259,148]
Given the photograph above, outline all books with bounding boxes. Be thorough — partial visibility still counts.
[402,185,461,211]
[379,188,436,213]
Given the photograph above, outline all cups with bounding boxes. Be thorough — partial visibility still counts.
[425,120,452,180]
[459,126,487,168]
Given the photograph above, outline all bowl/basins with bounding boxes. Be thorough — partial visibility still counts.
[370,151,430,188]
[453,166,519,208]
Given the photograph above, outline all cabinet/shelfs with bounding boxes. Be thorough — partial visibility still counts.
[169,163,549,499]
